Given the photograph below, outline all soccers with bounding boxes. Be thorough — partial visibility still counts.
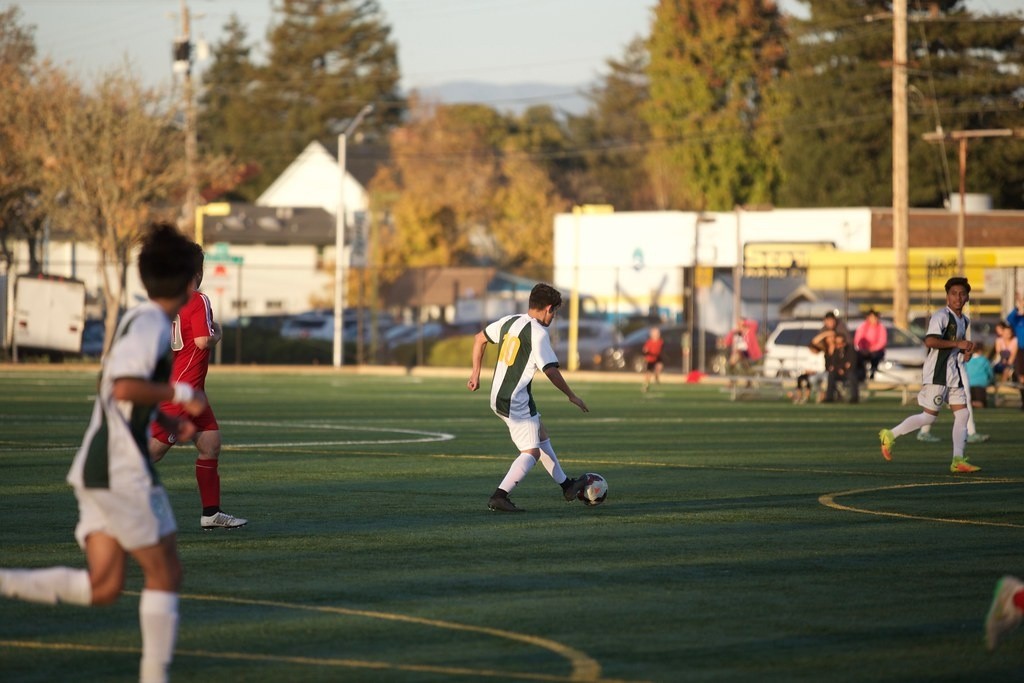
[577,473,609,506]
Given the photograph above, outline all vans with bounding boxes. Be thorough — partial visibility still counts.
[762,318,929,383]
[544,318,624,368]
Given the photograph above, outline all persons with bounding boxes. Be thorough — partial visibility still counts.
[640,327,664,392]
[988,288,1024,412]
[0,219,198,682]
[982,574,1024,654]
[854,308,887,383]
[964,339,994,408]
[467,282,590,512]
[808,310,854,370]
[819,334,866,405]
[723,316,762,383]
[879,277,989,473]
[147,242,248,530]
[794,329,848,405]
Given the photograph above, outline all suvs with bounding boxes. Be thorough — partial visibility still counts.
[231,306,446,357]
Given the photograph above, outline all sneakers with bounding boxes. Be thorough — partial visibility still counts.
[917,433,940,442]
[201,511,247,531]
[950,461,981,473]
[564,475,587,501]
[984,576,1024,650]
[488,497,522,512]
[967,433,990,443]
[879,428,895,461]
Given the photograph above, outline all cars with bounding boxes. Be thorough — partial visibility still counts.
[602,324,721,371]
